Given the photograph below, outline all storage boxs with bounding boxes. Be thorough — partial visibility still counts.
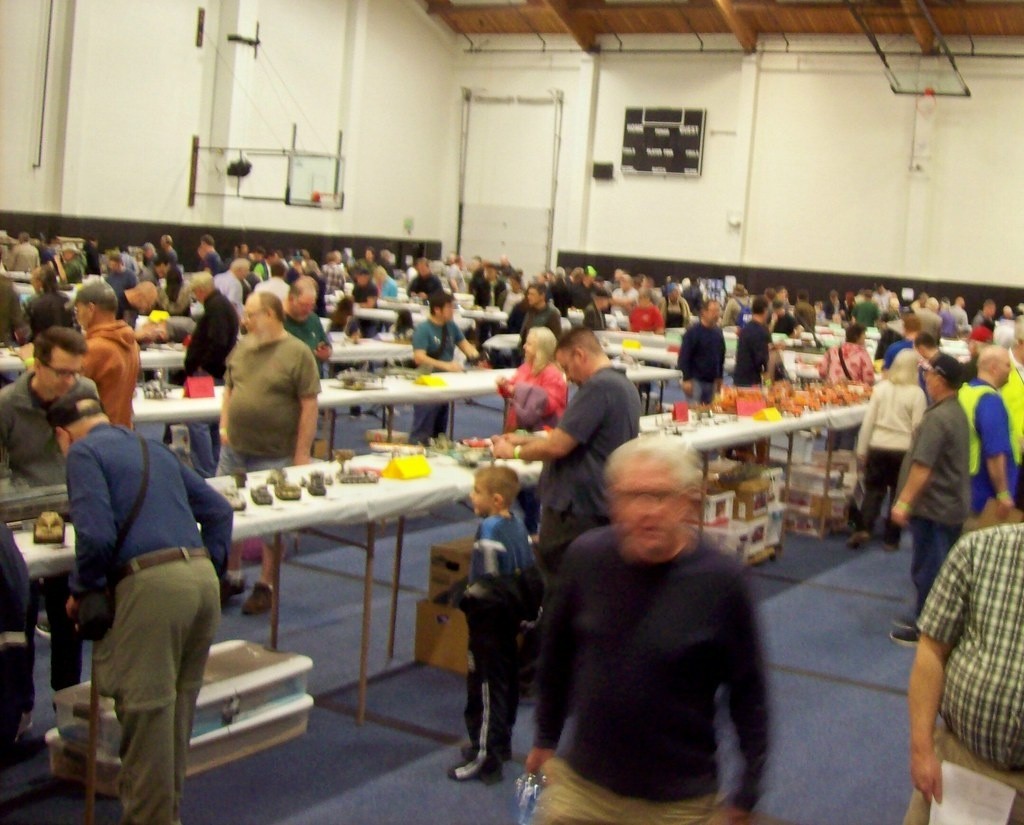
[758,461,790,505]
[732,474,769,520]
[785,509,828,537]
[794,460,845,490]
[691,483,734,526]
[784,486,850,516]
[704,513,772,560]
[426,536,478,605]
[410,598,475,673]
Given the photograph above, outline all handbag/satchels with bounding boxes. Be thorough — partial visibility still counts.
[77,592,111,639]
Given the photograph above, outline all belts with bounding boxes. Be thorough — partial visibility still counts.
[120,549,209,574]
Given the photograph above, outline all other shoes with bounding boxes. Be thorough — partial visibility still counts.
[461,742,514,760]
[243,582,272,615]
[894,616,916,630]
[448,759,500,780]
[220,578,245,602]
[845,531,868,548]
[881,539,899,552]
[890,627,917,646]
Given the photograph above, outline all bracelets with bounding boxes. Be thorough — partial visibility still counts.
[996,491,1010,498]
[514,445,520,459]
[221,428,227,432]
[896,501,913,512]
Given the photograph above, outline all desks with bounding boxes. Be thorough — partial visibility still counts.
[0,272,1011,825]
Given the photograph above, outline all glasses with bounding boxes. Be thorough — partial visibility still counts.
[43,364,80,379]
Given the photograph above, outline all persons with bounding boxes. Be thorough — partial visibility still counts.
[492,327,640,714]
[0,225,1023,743]
[51,393,232,825]
[888,352,969,645]
[0,517,37,744]
[957,345,1015,529]
[447,466,535,785]
[517,438,769,824]
[898,519,1024,825]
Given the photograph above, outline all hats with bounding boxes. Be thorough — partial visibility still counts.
[57,242,79,254]
[64,281,117,308]
[930,352,964,386]
[967,326,993,343]
[48,389,106,438]
